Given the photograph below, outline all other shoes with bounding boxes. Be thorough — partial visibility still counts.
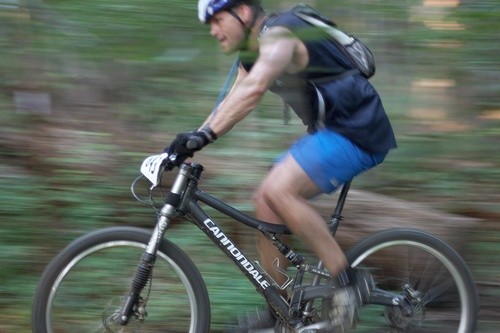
[331,267,373,332]
[249,311,275,332]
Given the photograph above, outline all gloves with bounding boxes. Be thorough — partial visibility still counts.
[165,131,210,170]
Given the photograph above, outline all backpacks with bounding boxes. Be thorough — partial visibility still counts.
[259,3,376,85]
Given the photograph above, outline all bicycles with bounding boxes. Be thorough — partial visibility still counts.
[31,141,480,333]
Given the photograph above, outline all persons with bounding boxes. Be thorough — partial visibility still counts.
[139,0,400,333]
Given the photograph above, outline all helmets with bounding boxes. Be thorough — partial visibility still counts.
[197,0,232,24]
